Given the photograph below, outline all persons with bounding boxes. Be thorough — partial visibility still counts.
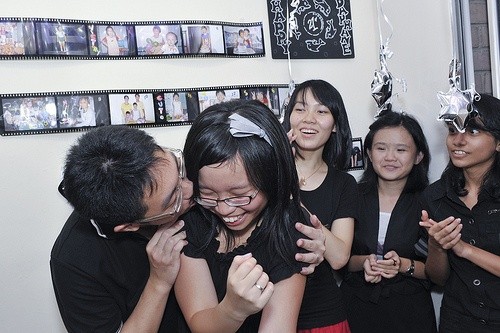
[145,26,179,54]
[75,96,97,127]
[19,101,36,121]
[282,81,360,333]
[121,94,146,124]
[170,93,184,120]
[56,26,67,52]
[174,98,314,333]
[49,127,328,333]
[101,25,122,56]
[338,113,438,333]
[215,90,268,106]
[0,26,8,41]
[235,29,253,48]
[200,27,211,48]
[416,94,500,333]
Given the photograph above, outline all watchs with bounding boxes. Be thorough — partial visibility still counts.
[406,259,415,276]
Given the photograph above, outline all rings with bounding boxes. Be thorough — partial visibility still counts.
[256,283,264,291]
[392,259,397,266]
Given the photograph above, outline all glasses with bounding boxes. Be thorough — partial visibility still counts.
[135,147,186,223]
[193,190,259,207]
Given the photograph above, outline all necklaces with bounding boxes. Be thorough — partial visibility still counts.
[296,162,324,186]
